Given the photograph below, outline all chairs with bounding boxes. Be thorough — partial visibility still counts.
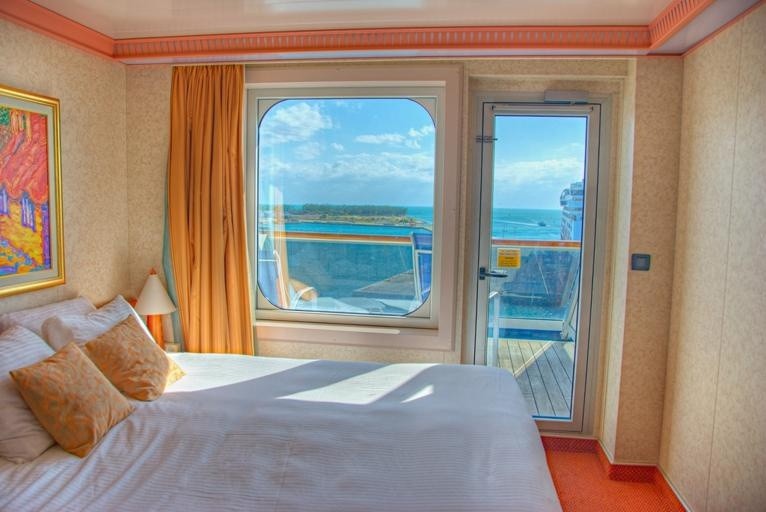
[256,249,320,309]
[409,231,504,366]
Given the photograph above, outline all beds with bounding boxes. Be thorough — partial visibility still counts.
[0,298,563,512]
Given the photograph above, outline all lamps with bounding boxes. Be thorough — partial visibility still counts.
[136,268,179,353]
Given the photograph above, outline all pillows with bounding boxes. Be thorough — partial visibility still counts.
[0,295,186,463]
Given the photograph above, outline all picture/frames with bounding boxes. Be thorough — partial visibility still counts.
[0,85,68,297]
